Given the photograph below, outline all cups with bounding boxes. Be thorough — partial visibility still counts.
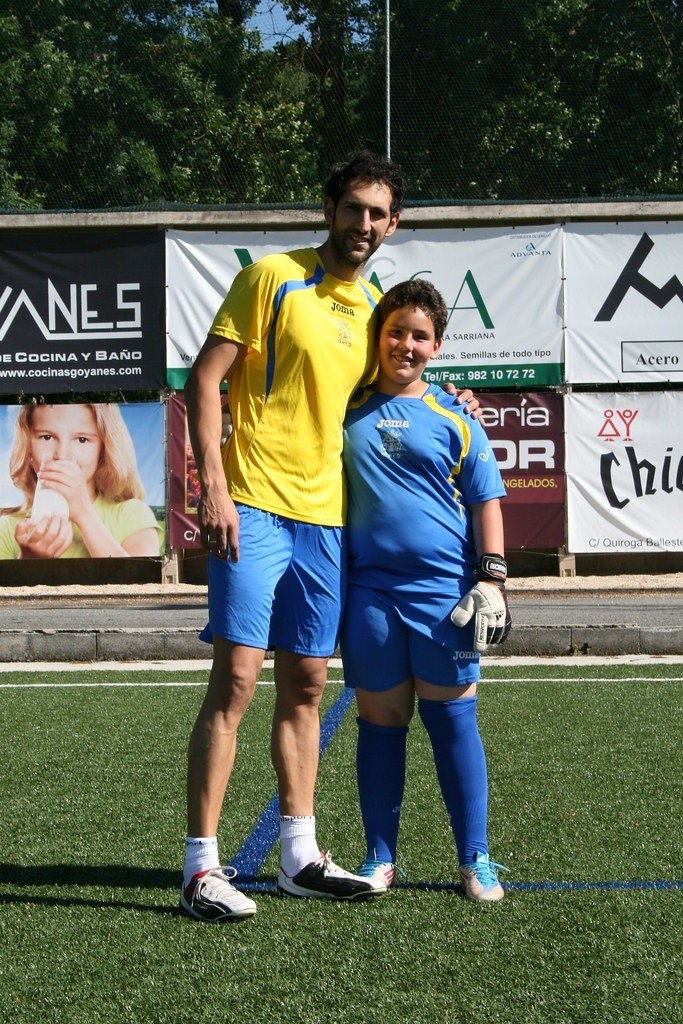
[29,451,79,534]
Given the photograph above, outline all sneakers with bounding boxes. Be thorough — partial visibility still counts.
[179,869,257,921]
[460,853,504,904]
[277,853,386,901]
[356,860,396,891]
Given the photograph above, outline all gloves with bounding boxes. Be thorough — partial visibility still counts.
[450,553,513,652]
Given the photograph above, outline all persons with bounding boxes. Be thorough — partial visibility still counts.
[0,402,164,559]
[179,159,485,922]
[338,279,512,903]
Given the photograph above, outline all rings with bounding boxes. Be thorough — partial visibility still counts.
[209,541,216,543]
